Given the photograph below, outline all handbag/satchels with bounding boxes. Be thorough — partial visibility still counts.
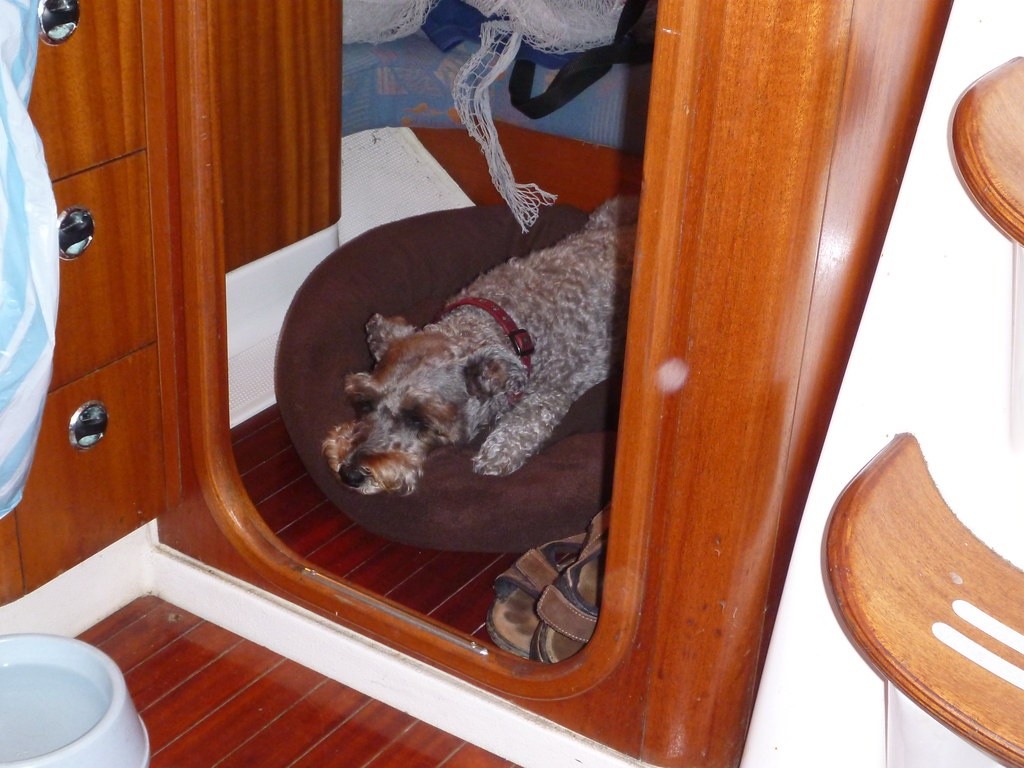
[421,0,654,120]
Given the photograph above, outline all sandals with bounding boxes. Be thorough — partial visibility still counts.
[486,532,587,660]
[531,501,610,665]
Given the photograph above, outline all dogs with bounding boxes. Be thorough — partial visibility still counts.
[321,192,642,498]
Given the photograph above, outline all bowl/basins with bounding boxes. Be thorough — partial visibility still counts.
[0,634,150,768]
